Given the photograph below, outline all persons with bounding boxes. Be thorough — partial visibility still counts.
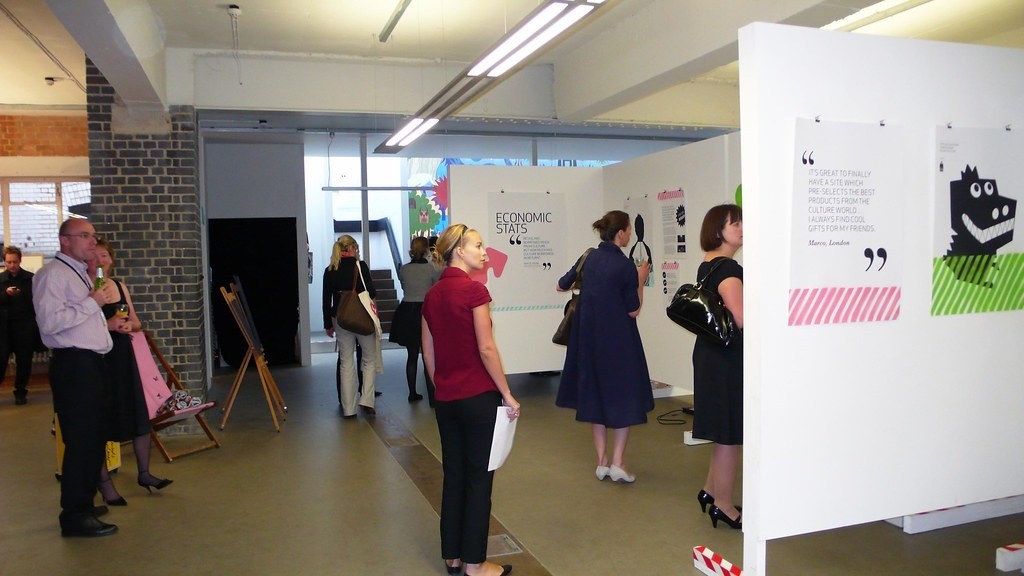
[690,203,743,528]
[427,236,447,282]
[0,246,37,405]
[83,239,173,506]
[319,234,385,417]
[421,224,520,576]
[29,218,120,538]
[556,210,649,482]
[388,236,438,408]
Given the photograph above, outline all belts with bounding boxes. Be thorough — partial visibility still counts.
[52,348,92,353]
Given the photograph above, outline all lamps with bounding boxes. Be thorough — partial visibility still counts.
[373,1,609,155]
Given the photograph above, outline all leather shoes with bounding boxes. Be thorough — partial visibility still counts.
[89,505,108,518]
[61,516,118,538]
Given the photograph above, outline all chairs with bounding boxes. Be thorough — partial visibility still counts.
[134,332,222,462]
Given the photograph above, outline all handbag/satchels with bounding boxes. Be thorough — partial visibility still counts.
[667,258,739,347]
[336,259,375,336]
[356,259,383,341]
[551,248,596,345]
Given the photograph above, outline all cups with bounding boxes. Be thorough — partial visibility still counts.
[116,303,128,316]
[636,256,644,268]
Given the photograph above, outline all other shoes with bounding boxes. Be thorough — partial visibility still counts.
[360,390,383,396]
[14,392,28,406]
[464,565,512,576]
[363,405,376,416]
[445,559,463,576]
[343,414,357,419]
[409,394,423,402]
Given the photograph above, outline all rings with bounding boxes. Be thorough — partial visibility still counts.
[514,412,518,416]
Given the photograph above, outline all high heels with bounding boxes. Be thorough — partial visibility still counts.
[98,478,128,507]
[595,465,610,481]
[709,504,742,529]
[610,464,635,482]
[697,489,742,513]
[138,471,174,494]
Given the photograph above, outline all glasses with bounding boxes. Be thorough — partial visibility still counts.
[352,245,360,253]
[65,232,98,241]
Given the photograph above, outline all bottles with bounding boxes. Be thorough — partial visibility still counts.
[93,264,106,291]
